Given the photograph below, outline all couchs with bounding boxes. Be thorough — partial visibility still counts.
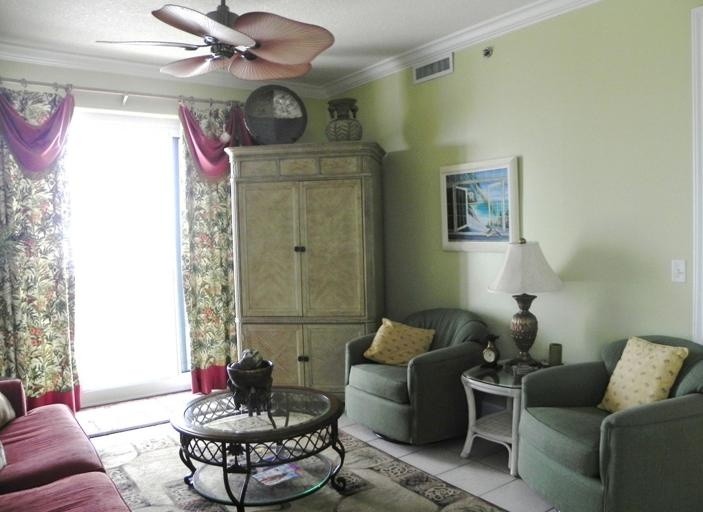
[0,378,131,512]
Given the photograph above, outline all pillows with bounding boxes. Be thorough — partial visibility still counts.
[0,440,8,470]
[597,336,689,413]
[0,392,16,428]
[363,317,437,366]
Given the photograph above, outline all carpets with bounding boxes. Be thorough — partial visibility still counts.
[88,420,510,512]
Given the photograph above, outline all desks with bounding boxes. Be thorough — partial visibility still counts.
[460,359,549,476]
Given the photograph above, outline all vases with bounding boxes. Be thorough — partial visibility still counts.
[326,95,362,141]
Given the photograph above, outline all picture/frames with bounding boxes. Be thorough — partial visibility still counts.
[440,156,520,252]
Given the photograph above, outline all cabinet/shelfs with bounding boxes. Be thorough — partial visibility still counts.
[224,140,386,404]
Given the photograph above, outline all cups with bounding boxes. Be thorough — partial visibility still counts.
[548,342,562,369]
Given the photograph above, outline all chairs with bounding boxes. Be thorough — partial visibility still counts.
[345,309,490,446]
[518,336,703,512]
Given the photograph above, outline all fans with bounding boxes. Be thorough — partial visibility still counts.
[96,0,334,81]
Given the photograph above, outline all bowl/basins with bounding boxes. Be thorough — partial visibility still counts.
[226,357,274,393]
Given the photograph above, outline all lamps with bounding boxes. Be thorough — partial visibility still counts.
[487,238,562,377]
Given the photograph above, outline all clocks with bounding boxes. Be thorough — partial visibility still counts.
[480,334,503,369]
